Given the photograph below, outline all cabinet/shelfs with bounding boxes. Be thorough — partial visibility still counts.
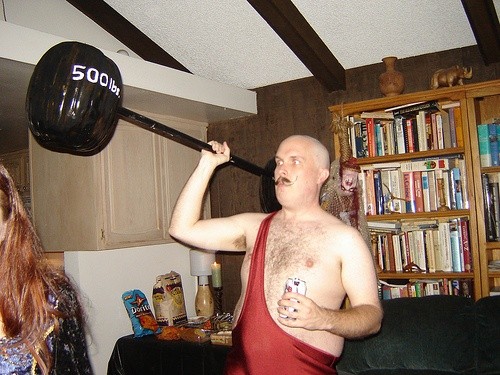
[0,107,212,254]
[328,80,500,309]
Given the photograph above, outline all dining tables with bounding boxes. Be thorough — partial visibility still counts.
[107,334,231,375]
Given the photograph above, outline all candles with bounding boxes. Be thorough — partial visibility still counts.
[211,262,222,287]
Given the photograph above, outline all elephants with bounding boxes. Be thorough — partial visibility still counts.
[430,63,472,89]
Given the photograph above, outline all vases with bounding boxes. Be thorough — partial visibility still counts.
[379,57,405,97]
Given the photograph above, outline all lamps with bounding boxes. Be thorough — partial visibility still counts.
[190,250,215,317]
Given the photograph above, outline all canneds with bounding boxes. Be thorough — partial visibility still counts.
[279,278,307,322]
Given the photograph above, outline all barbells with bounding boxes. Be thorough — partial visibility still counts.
[24,42,322,213]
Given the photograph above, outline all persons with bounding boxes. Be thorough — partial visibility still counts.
[0,162,94,375]
[167,134,385,375]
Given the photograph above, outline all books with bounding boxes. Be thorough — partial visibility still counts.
[354,217,472,273]
[476,117,500,168]
[377,274,475,301]
[481,172,500,244]
[345,153,468,216]
[342,99,464,159]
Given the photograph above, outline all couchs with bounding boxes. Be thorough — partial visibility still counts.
[335,295,500,375]
